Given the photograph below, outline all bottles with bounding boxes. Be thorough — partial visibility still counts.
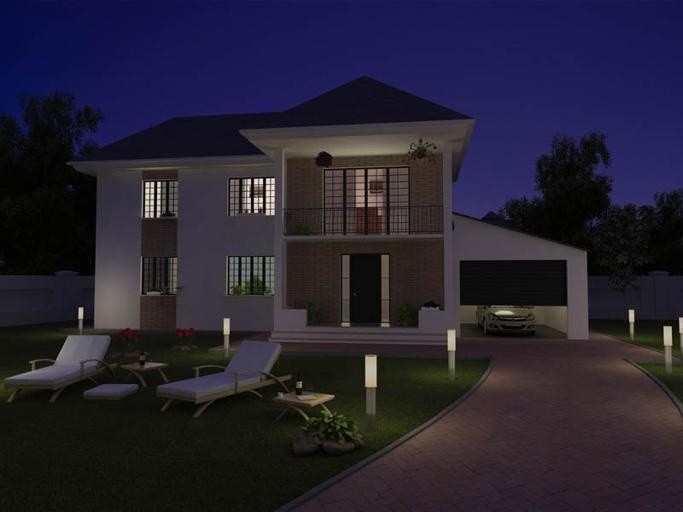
[296,371,303,396]
[139,348,145,369]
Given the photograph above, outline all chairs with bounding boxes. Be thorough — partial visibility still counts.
[3,334,119,404]
[157,339,292,418]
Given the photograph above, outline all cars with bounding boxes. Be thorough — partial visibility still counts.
[475,305,536,337]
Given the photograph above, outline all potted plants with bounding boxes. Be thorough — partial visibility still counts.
[396,304,416,326]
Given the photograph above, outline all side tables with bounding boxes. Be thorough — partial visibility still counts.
[121,362,169,387]
[273,391,335,426]
